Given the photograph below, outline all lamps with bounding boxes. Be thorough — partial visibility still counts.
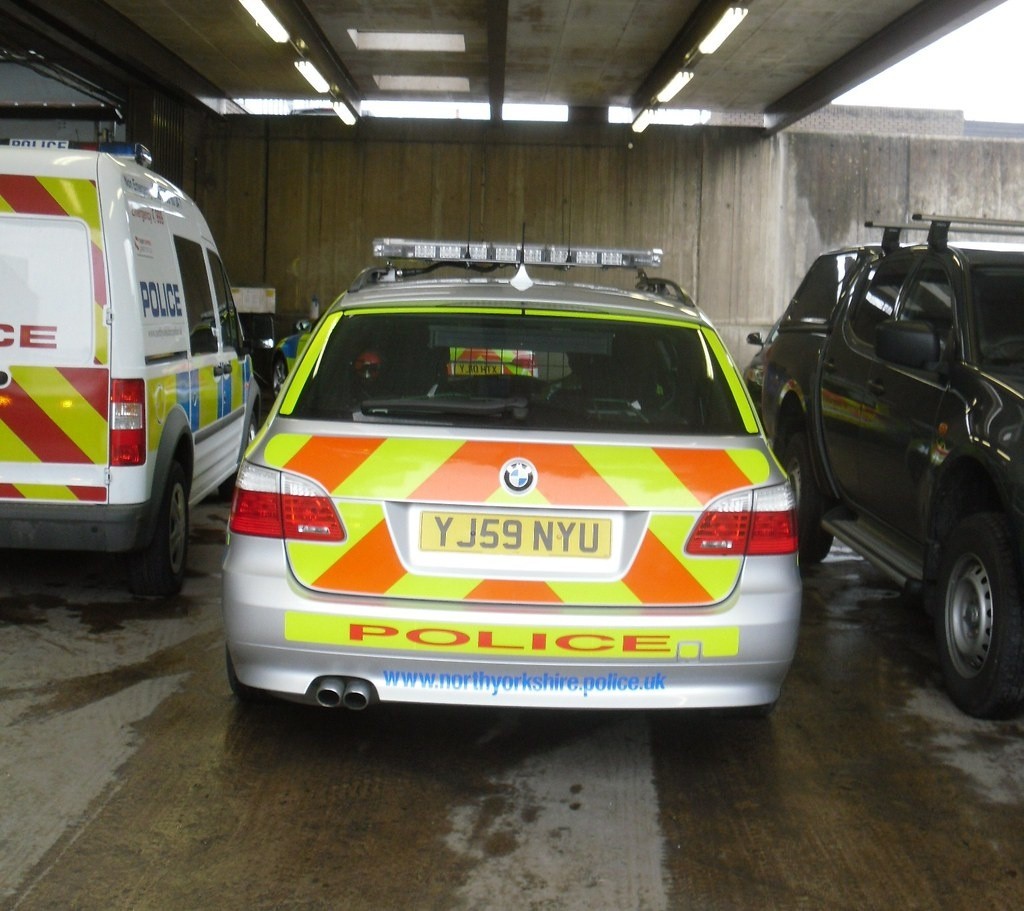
[695,4,749,55]
[295,57,331,95]
[238,0,290,44]
[631,105,658,134]
[329,99,357,126]
[655,68,694,104]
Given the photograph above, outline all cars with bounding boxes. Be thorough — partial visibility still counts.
[218,232,805,723]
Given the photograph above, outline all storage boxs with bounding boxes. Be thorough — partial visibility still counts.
[230,287,276,314]
[239,315,283,345]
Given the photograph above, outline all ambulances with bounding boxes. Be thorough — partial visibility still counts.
[1,134,266,601]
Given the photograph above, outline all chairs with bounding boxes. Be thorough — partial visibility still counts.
[366,344,449,399]
[552,354,658,414]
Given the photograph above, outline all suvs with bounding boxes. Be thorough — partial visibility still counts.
[741,214,1024,721]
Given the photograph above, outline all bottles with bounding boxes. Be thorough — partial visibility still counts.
[309,295,319,319]
